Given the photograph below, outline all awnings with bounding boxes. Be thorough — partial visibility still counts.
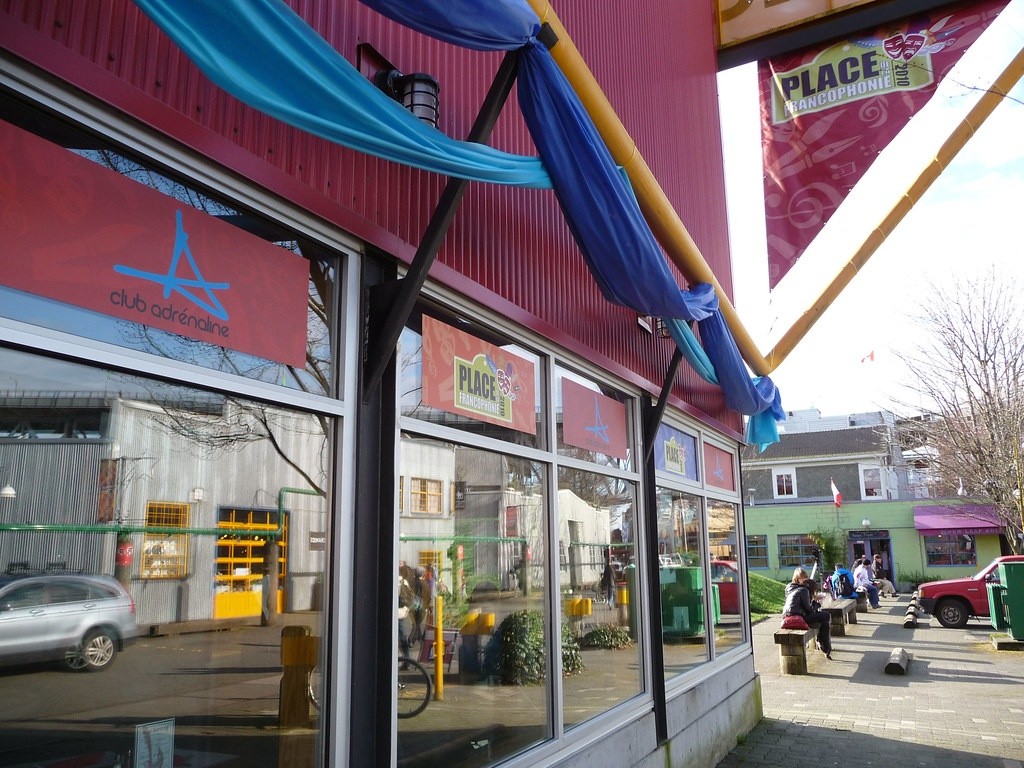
[912,504,1024,536]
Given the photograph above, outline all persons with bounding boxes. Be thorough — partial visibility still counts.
[406,566,428,647]
[785,567,826,611]
[398,566,415,670]
[601,559,618,611]
[783,578,833,660]
[832,563,859,604]
[853,559,883,609]
[425,567,435,608]
[871,553,899,598]
[850,551,862,574]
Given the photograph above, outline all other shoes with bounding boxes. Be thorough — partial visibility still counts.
[892,593,899,597]
[878,592,887,598]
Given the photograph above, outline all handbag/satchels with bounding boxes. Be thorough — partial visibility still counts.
[781,615,810,630]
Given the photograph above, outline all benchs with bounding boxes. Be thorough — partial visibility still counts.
[820,597,858,637]
[774,620,823,675]
[853,580,884,612]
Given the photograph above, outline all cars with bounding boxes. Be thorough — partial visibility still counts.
[710,560,740,614]
[917,555,1024,629]
[0,574,138,672]
[611,552,684,584]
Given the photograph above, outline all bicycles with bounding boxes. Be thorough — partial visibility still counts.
[310,657,433,718]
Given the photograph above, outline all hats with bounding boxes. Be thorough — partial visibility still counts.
[864,559,871,565]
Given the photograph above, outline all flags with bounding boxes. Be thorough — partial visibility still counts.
[831,479,842,508]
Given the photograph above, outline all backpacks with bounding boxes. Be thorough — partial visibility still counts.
[836,573,855,597]
